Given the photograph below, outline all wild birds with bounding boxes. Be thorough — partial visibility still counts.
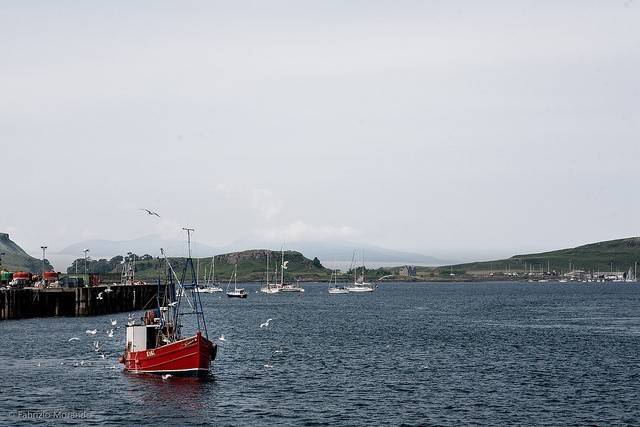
[271,346,283,357]
[106,327,117,338]
[218,334,227,342]
[96,292,104,300]
[93,342,100,349]
[35,362,42,367]
[162,374,172,380]
[127,320,136,327]
[162,335,171,343]
[259,319,273,329]
[104,285,113,294]
[109,317,117,327]
[127,313,136,320]
[97,352,110,357]
[105,366,116,370]
[167,298,178,308]
[280,261,289,270]
[79,360,86,366]
[68,337,81,343]
[73,362,79,367]
[139,208,161,218]
[264,362,274,373]
[84,329,99,335]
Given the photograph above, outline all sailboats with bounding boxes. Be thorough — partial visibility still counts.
[489,260,638,281]
[328,255,349,293]
[206,255,223,291]
[261,241,300,293]
[190,255,211,293]
[226,263,248,297]
[347,249,378,292]
[119,227,218,374]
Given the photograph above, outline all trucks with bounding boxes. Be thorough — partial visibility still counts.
[0,269,125,287]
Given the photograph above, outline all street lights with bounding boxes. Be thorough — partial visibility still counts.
[83,249,90,274]
[41,246,47,278]
